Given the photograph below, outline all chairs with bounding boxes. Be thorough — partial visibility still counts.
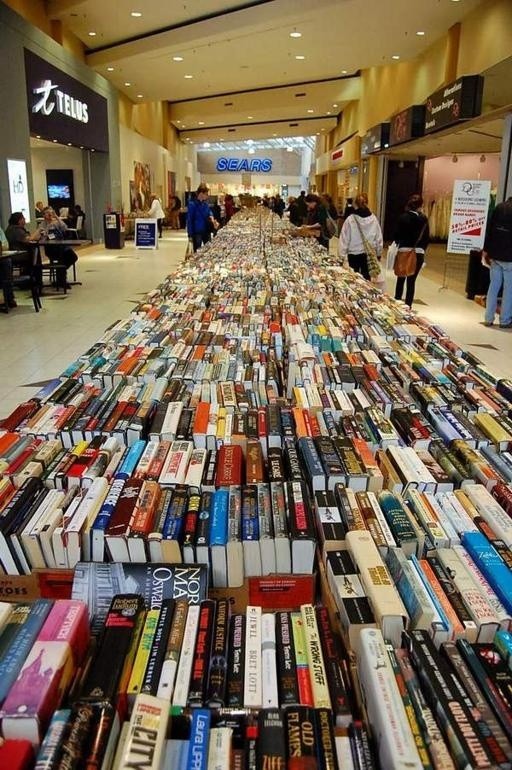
[1,228,95,316]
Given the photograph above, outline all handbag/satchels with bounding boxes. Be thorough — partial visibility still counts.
[395,250,416,278]
[323,215,335,240]
[364,241,381,278]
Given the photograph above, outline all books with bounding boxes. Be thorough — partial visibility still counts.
[216,207,304,234]
[147,231,380,295]
[0,289,512,769]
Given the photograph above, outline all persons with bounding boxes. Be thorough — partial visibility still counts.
[44,207,78,286]
[71,205,85,226]
[4,212,43,284]
[34,202,44,228]
[337,193,384,282]
[259,191,355,250]
[0,229,17,307]
[482,197,512,328]
[390,194,429,310]
[147,183,235,252]
